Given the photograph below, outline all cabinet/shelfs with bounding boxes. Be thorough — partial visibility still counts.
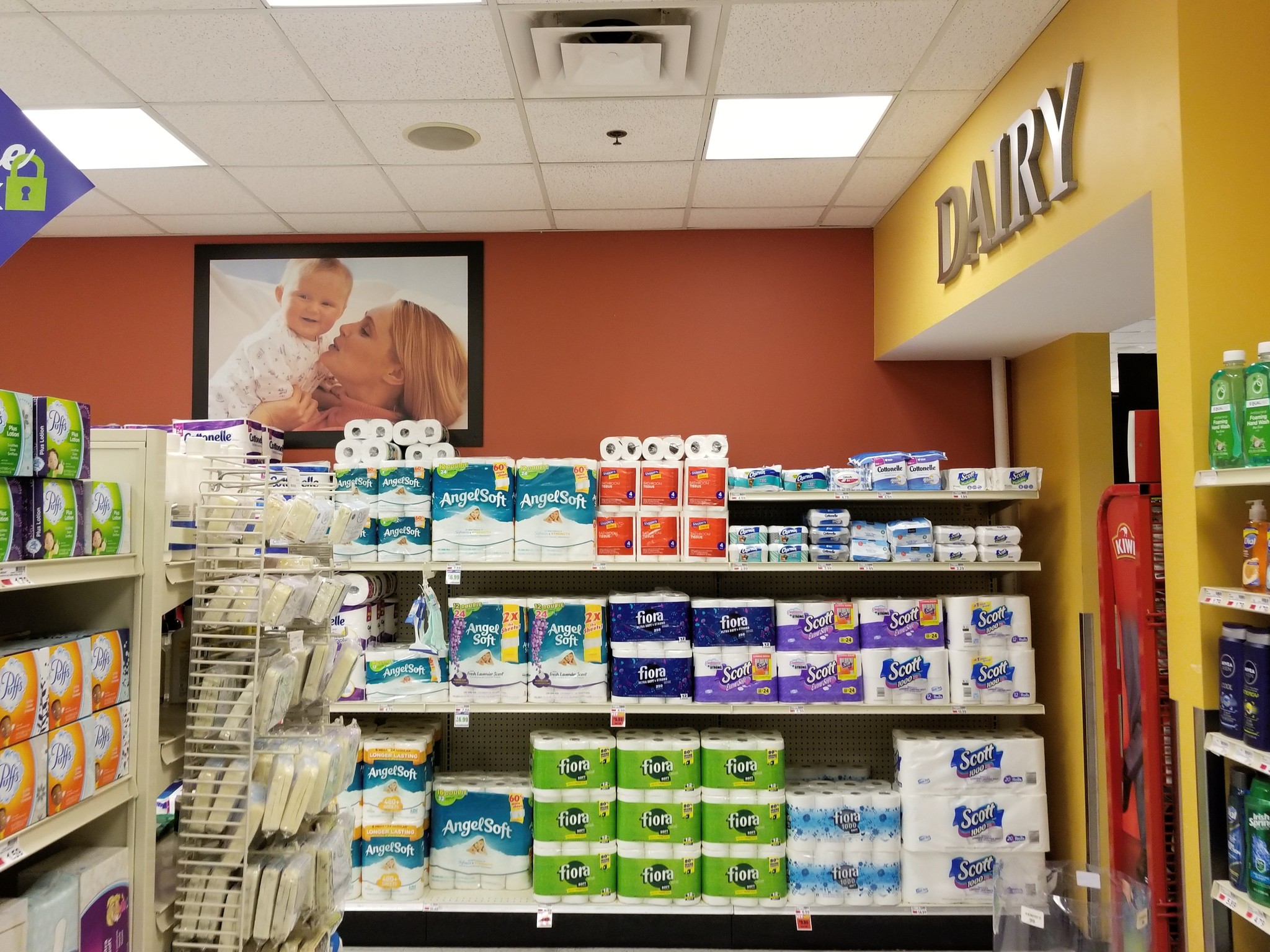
[1189,457,1270,940]
[0,430,147,952]
[326,486,1046,924]
[145,423,238,952]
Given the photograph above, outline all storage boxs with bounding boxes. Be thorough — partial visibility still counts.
[0,387,132,952]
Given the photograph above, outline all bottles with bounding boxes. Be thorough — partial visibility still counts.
[1243,341,1270,468]
[1208,350,1247,469]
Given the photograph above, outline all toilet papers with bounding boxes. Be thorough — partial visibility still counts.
[335,715,1049,907]
[329,418,730,564]
[325,572,1036,705]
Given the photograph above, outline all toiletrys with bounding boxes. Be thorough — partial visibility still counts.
[1225,766,1270,908]
[1207,340,1270,468]
[1216,620,1269,751]
[1241,498,1270,593]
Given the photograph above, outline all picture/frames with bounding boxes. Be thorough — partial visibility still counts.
[191,238,487,449]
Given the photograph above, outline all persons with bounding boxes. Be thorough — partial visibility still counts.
[248,298,467,431]
[208,258,353,421]
